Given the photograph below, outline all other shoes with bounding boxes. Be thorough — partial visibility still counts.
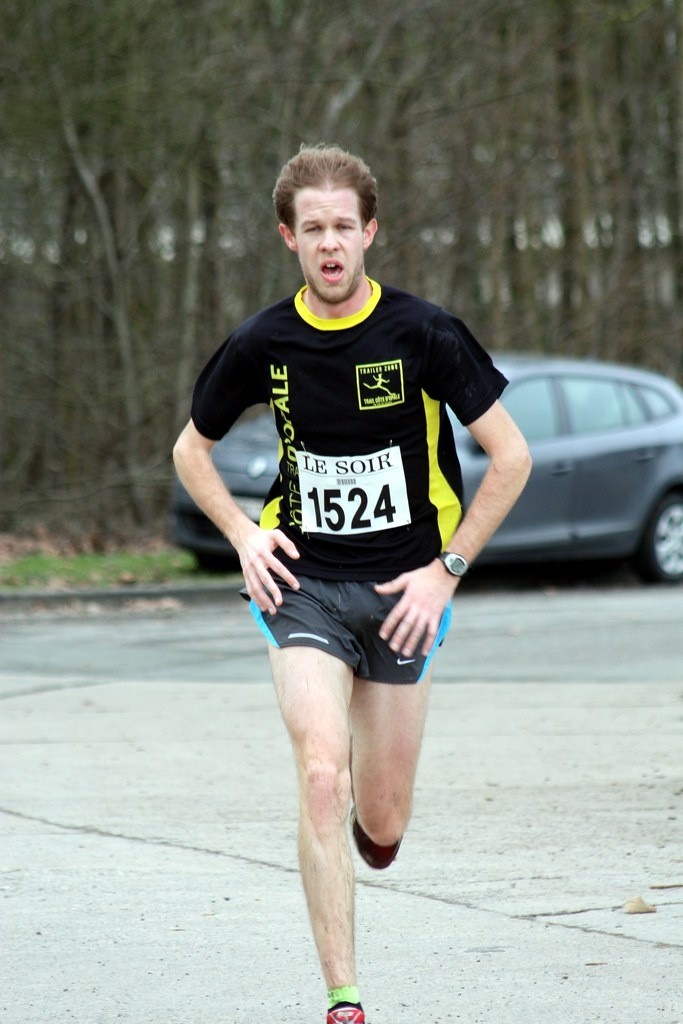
[326,1001,364,1024]
[346,732,404,870]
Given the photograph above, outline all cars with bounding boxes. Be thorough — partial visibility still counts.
[167,351,683,588]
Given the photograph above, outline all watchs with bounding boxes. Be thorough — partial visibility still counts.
[438,552,469,577]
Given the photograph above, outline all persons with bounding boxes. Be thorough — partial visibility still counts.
[172,147,533,1024]
